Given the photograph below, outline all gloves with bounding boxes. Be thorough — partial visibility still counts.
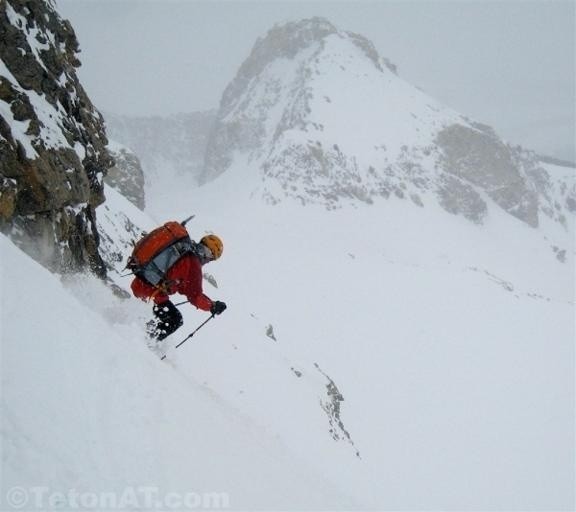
[213,300,227,315]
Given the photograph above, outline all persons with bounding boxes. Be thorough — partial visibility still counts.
[130,235,225,348]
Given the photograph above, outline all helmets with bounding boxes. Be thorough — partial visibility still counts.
[200,235,224,260]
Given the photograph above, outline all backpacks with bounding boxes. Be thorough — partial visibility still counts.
[126,221,192,287]
